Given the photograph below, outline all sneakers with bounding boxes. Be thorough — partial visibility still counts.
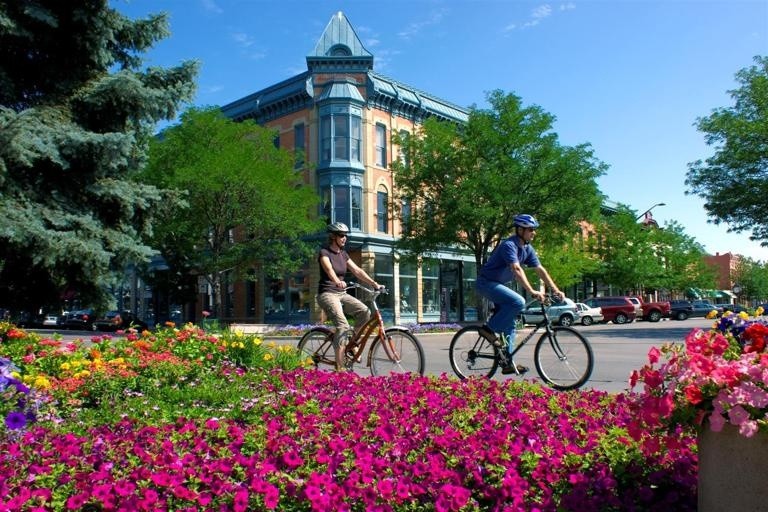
[346,344,361,363]
[478,326,501,347]
[501,366,529,374]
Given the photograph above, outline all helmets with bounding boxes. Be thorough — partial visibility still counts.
[326,222,351,234]
[513,214,540,229]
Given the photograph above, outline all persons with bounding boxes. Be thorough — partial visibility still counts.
[422,288,429,305]
[375,287,387,308]
[399,292,412,312]
[475,213,566,375]
[317,222,387,373]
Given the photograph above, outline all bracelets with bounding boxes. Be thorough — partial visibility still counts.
[372,282,377,286]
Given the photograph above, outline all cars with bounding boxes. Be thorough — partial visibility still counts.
[2,305,147,335]
[374,301,480,325]
[127,303,310,324]
[514,294,767,329]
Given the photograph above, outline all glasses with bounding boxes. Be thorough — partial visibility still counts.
[333,233,348,237]
[529,228,536,232]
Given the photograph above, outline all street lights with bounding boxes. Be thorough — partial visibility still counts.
[296,279,425,378]
[635,201,665,221]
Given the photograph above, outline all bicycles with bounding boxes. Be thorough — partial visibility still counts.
[447,292,594,391]
